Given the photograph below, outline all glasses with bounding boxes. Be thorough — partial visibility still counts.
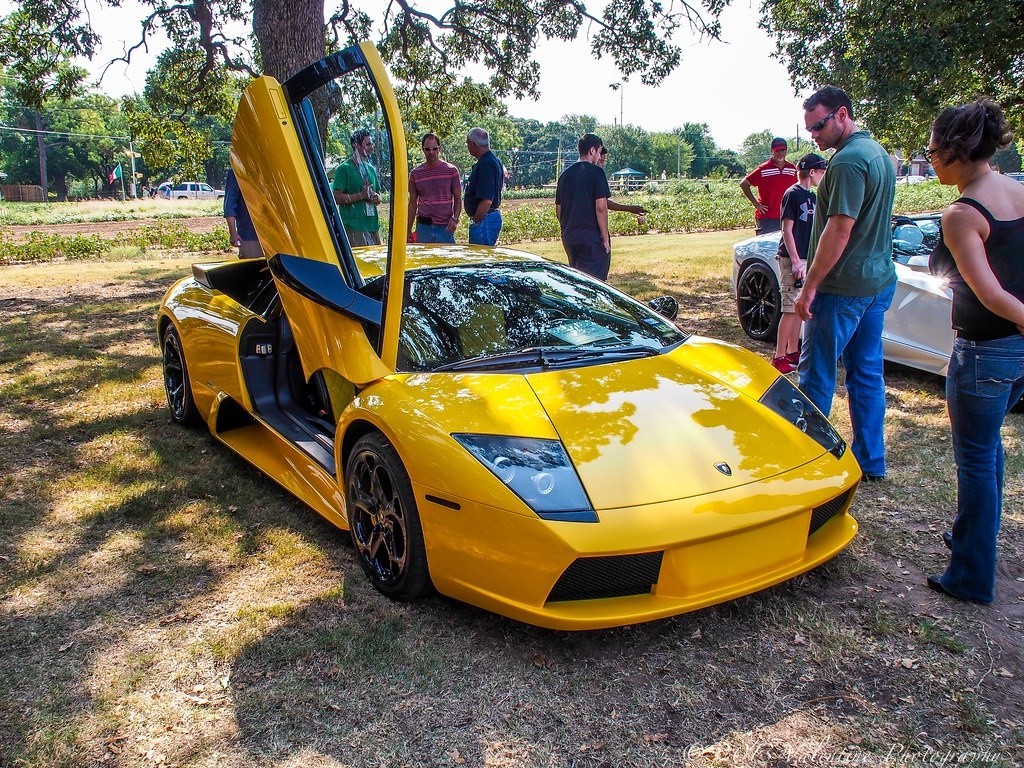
[807,104,847,131]
[924,141,956,162]
[423,145,440,151]
[807,160,828,166]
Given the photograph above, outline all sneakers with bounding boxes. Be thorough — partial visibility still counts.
[771,356,796,375]
[785,351,800,368]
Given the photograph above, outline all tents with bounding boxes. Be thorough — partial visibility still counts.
[613,166,646,190]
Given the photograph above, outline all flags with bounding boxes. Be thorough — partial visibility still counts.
[503,163,510,179]
[109,163,121,184]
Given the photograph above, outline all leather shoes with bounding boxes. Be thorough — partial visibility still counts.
[943,531,954,547]
[927,575,946,592]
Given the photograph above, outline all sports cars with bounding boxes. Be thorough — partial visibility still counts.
[731,213,1024,413]
[157,39,863,630]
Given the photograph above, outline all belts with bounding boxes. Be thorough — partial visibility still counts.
[488,209,499,213]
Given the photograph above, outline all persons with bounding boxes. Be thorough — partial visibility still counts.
[615,174,643,191]
[923,95,1024,604]
[142,184,155,197]
[555,134,612,282]
[223,168,264,259]
[740,138,798,236]
[408,133,462,244]
[596,147,645,216]
[165,185,171,200]
[661,170,666,183]
[332,129,382,247]
[771,152,826,375]
[464,127,503,247]
[794,88,898,482]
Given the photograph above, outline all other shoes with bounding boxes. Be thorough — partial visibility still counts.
[862,476,884,481]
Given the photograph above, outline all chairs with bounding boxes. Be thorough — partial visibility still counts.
[316,368,358,427]
[893,224,924,265]
[456,302,509,357]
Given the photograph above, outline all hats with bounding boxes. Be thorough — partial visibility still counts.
[771,138,787,152]
[799,153,828,170]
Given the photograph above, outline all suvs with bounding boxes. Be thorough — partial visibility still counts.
[1006,171,1024,185]
[157,181,226,201]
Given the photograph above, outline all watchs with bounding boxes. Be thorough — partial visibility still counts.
[451,217,459,222]
